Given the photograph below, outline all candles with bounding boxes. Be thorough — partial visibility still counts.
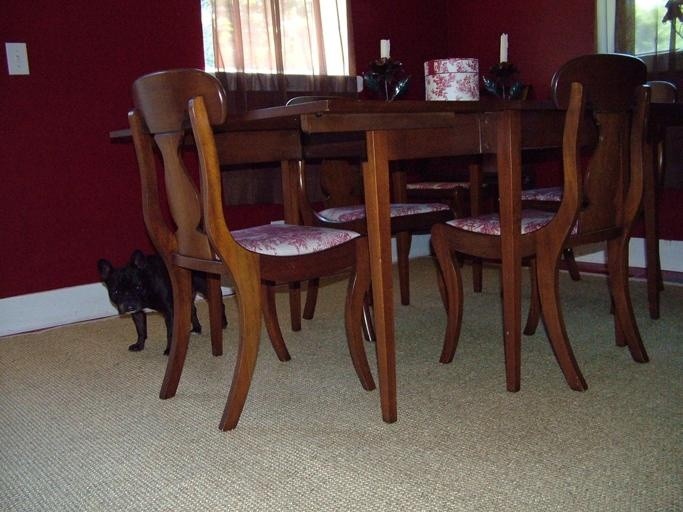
[499,33,509,63]
[380,38,391,60]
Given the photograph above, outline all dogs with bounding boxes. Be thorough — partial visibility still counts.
[95,248,229,357]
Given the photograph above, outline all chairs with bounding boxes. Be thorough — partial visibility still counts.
[128,68,375,433]
[391,162,534,292]
[284,95,456,341]
[430,53,652,393]
[499,79,680,315]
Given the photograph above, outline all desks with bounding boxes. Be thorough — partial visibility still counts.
[106,99,682,425]
